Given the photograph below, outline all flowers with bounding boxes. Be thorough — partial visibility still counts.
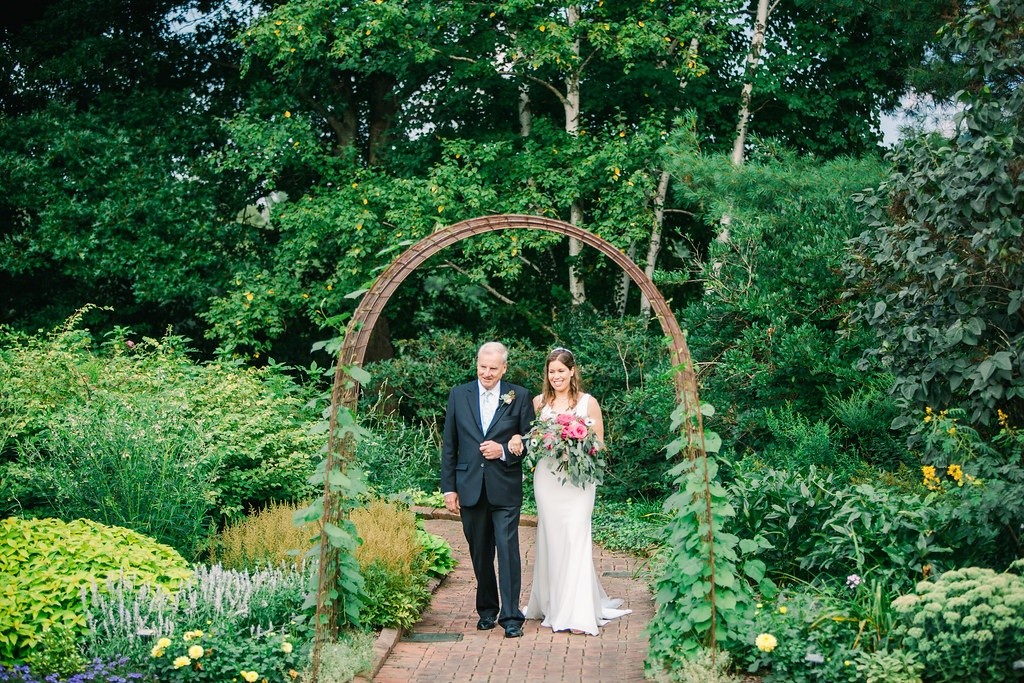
[525,413,608,485]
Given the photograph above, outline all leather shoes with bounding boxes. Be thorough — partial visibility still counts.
[477,616,498,630]
[505,624,524,638]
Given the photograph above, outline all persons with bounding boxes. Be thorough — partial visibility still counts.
[508,347,632,636]
[440,342,536,640]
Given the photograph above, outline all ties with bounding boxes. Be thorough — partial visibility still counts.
[481,391,495,436]
